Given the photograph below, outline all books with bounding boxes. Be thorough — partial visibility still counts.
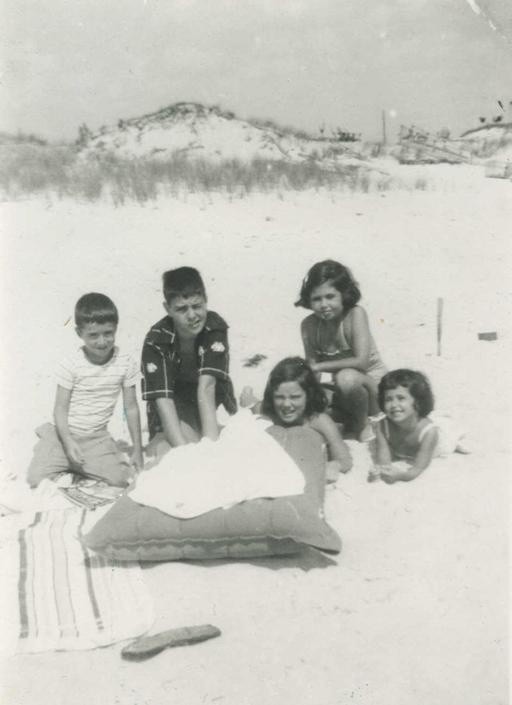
[59,479,125,512]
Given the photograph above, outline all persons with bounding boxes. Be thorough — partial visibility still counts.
[141,267,238,458]
[368,368,472,485]
[241,356,353,485]
[28,293,146,489]
[293,259,390,443]
